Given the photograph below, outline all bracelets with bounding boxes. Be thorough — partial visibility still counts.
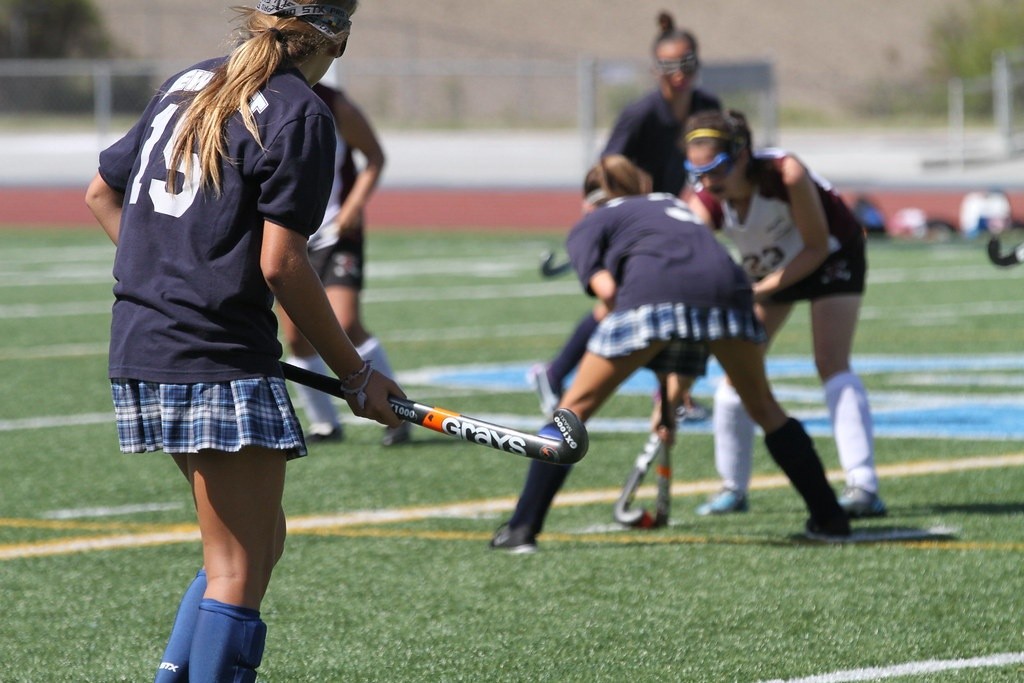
[341,361,372,409]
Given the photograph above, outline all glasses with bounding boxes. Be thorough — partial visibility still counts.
[682,154,733,185]
[655,52,701,79]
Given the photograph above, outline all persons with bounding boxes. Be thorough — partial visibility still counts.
[958,178,1011,237]
[85,1,413,683]
[488,8,888,552]
[287,80,412,448]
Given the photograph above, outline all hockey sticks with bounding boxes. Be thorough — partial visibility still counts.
[647,371,673,530]
[275,357,589,464]
[614,418,673,531]
[537,246,578,284]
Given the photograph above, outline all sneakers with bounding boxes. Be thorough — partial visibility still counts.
[695,490,751,519]
[303,425,345,446]
[381,421,412,448]
[839,485,887,520]
[805,517,852,542]
[489,523,538,557]
